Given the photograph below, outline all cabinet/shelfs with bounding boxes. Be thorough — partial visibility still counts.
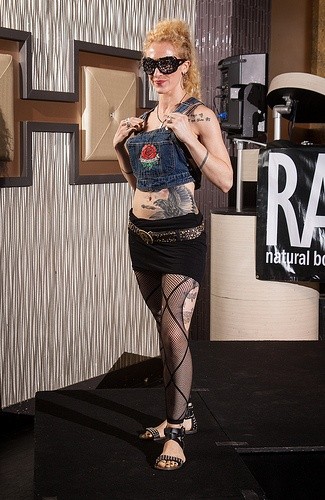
[210,208,320,341]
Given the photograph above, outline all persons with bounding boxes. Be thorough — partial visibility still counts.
[112,19,233,470]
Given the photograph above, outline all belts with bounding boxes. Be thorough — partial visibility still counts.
[128,219,204,244]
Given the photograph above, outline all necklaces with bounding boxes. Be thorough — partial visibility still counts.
[156,93,187,128]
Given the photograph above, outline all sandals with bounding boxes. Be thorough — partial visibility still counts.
[140,403,197,440]
[155,426,187,470]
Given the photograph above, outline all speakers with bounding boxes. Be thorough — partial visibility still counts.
[218,54,268,138]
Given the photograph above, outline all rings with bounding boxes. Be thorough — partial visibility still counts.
[126,121,130,129]
[168,117,172,123]
[126,118,130,121]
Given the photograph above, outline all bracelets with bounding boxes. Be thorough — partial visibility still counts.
[198,150,209,170]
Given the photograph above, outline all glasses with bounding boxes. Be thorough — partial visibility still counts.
[142,57,188,75]
